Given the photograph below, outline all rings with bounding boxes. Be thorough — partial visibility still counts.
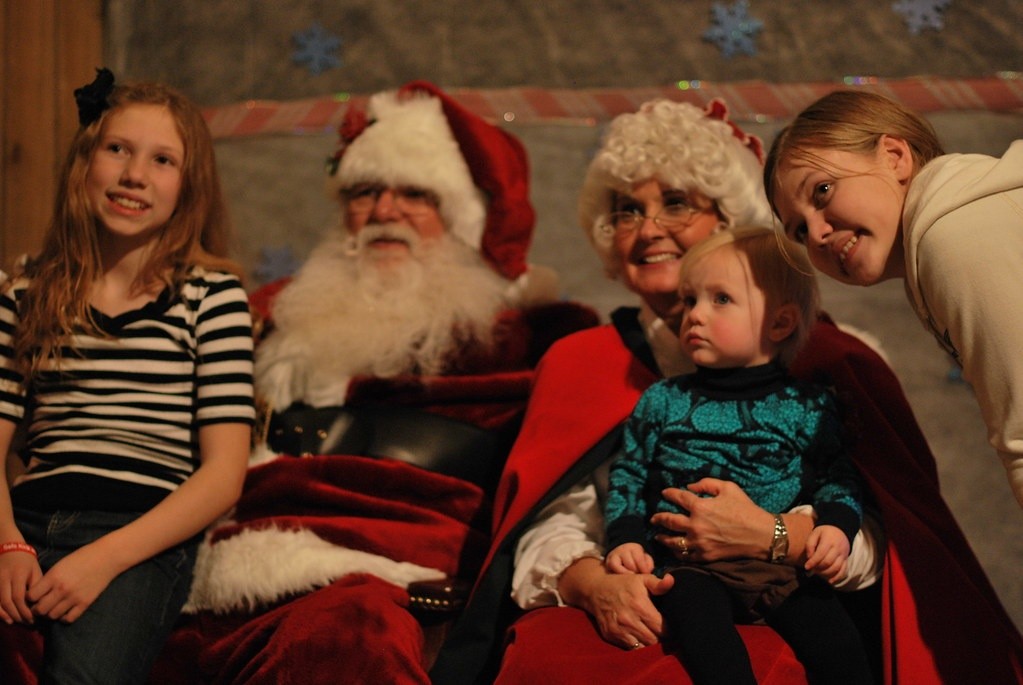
[631,641,640,648]
[682,539,688,555]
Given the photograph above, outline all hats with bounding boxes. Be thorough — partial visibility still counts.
[337,82,533,283]
[578,98,764,279]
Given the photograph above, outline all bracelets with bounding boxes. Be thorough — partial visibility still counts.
[0,541,37,556]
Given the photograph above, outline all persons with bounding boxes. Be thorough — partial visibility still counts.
[174,86,599,685]
[600,226,880,685]
[456,97,1023,685]
[0,70,258,685]
[763,90,1023,502]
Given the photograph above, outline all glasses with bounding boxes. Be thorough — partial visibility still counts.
[600,204,715,234]
[343,189,437,215]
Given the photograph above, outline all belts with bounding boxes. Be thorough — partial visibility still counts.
[265,405,503,487]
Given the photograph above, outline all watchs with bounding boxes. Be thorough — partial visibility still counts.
[772,513,789,561]
